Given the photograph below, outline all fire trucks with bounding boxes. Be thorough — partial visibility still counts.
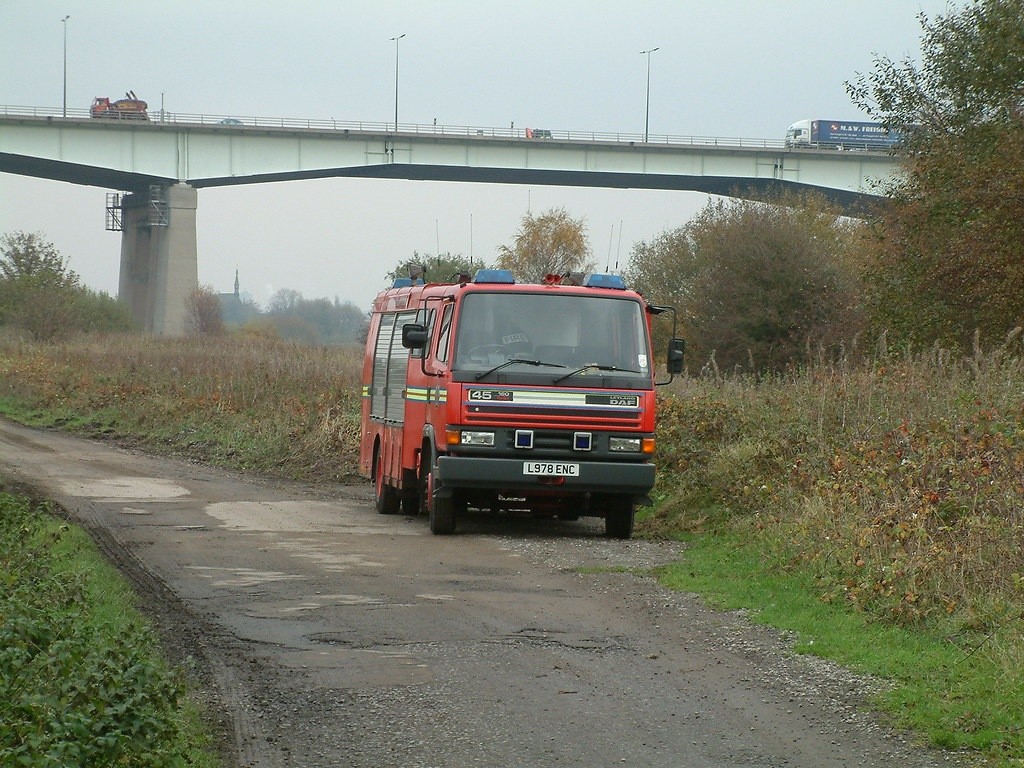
[357,213,684,536]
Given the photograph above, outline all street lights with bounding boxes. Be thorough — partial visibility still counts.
[391,33,406,132]
[61,15,71,119]
[639,48,658,144]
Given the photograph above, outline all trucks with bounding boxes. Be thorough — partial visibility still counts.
[89,96,148,119]
[786,119,932,151]
